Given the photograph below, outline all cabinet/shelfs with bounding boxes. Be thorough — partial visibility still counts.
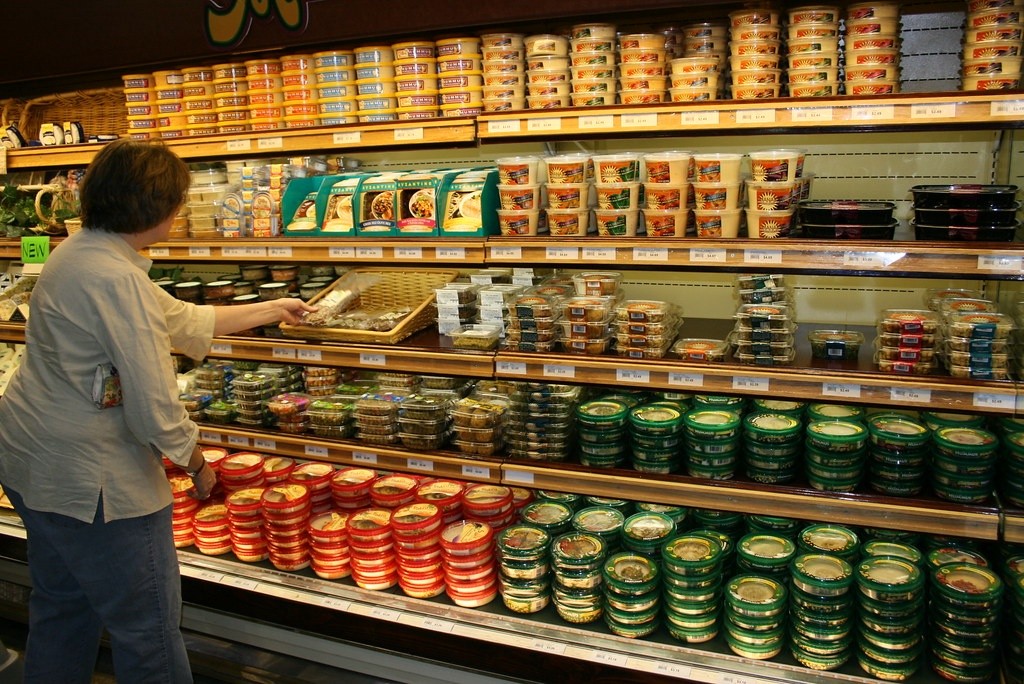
[1,93,1024,684]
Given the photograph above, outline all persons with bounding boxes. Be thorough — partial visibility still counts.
[0,137,319,684]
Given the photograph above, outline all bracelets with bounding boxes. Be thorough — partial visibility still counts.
[186,454,205,477]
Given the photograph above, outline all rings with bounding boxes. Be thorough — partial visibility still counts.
[206,494,210,498]
